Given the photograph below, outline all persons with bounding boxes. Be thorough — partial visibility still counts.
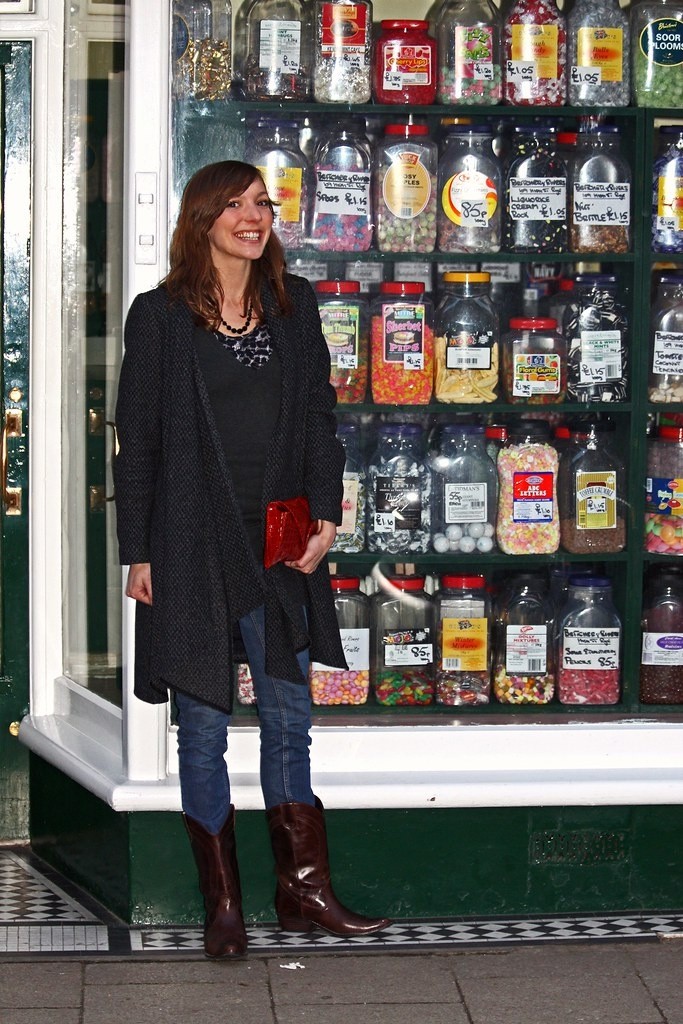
[111,160,393,959]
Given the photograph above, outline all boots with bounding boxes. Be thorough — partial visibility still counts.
[181,804,248,959]
[265,795,392,937]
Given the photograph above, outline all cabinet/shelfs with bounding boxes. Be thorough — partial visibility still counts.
[166,102,683,730]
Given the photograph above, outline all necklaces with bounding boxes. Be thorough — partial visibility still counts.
[220,297,253,334]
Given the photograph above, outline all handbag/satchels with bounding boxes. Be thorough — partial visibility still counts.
[264,496,318,569]
